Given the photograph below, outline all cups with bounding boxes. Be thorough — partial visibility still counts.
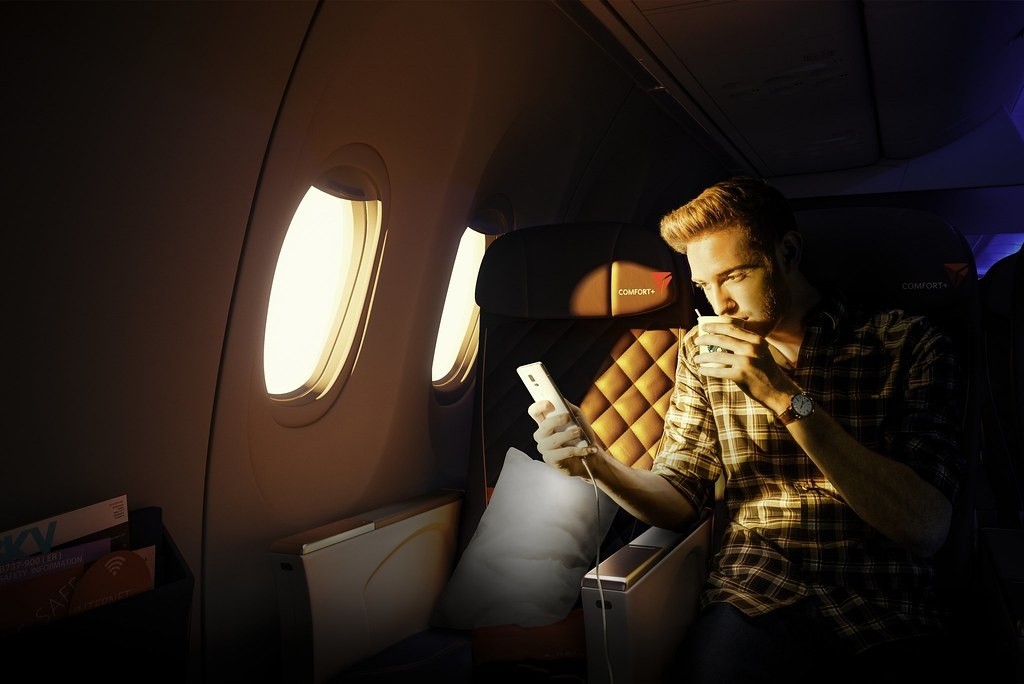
[698,316,746,368]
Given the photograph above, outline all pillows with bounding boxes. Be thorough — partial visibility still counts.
[431,447,618,632]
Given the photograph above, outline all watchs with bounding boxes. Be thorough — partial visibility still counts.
[774,391,816,427]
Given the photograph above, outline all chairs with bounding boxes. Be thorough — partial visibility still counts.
[266,206,1024,684]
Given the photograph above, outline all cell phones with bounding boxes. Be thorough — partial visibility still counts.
[515,361,591,450]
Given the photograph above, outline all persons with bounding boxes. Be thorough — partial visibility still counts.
[527,178,986,684]
